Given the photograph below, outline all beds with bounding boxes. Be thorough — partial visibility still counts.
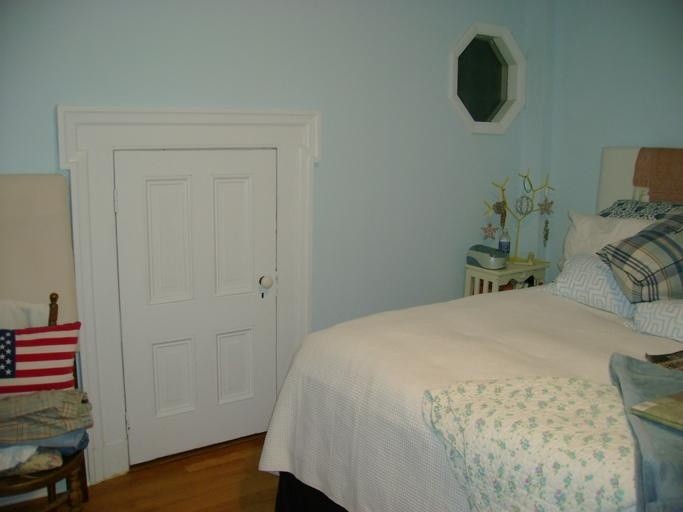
[268,143,683,512]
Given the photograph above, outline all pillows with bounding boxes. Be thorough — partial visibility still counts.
[1,320,82,397]
[550,186,683,341]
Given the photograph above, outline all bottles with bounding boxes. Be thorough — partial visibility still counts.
[499,228,510,264]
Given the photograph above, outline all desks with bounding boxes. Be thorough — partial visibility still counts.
[0,452,89,512]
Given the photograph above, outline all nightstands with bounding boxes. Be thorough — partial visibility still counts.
[461,257,549,297]
